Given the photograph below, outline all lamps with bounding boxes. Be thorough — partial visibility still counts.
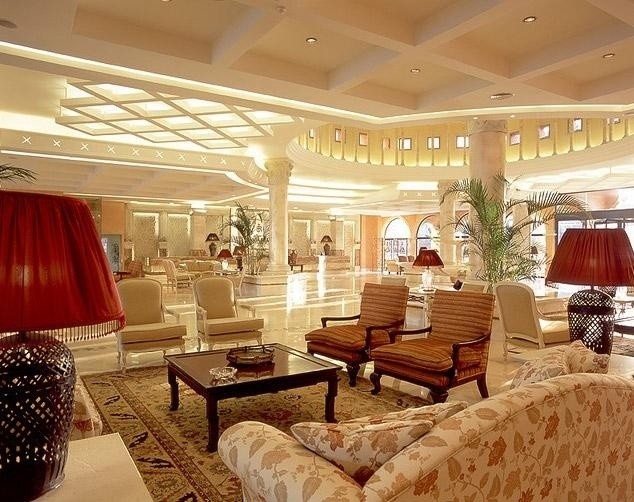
[205,233,246,271]
[0,186,125,502]
[413,249,444,288]
[597,286,616,298]
[321,235,332,255]
[158,241,168,258]
[545,228,634,353]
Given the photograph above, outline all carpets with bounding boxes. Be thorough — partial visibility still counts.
[81,364,434,502]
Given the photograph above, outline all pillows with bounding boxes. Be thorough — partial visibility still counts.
[291,402,468,481]
[570,339,610,375]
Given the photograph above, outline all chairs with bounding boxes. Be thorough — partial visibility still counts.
[288,250,303,272]
[111,258,221,288]
[408,256,414,262]
[399,256,407,262]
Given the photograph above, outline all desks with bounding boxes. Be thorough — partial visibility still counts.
[613,298,633,312]
[388,262,414,275]
[614,318,634,337]
[296,256,350,270]
[1,432,152,502]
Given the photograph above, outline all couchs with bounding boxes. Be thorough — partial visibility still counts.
[215,339,634,502]
